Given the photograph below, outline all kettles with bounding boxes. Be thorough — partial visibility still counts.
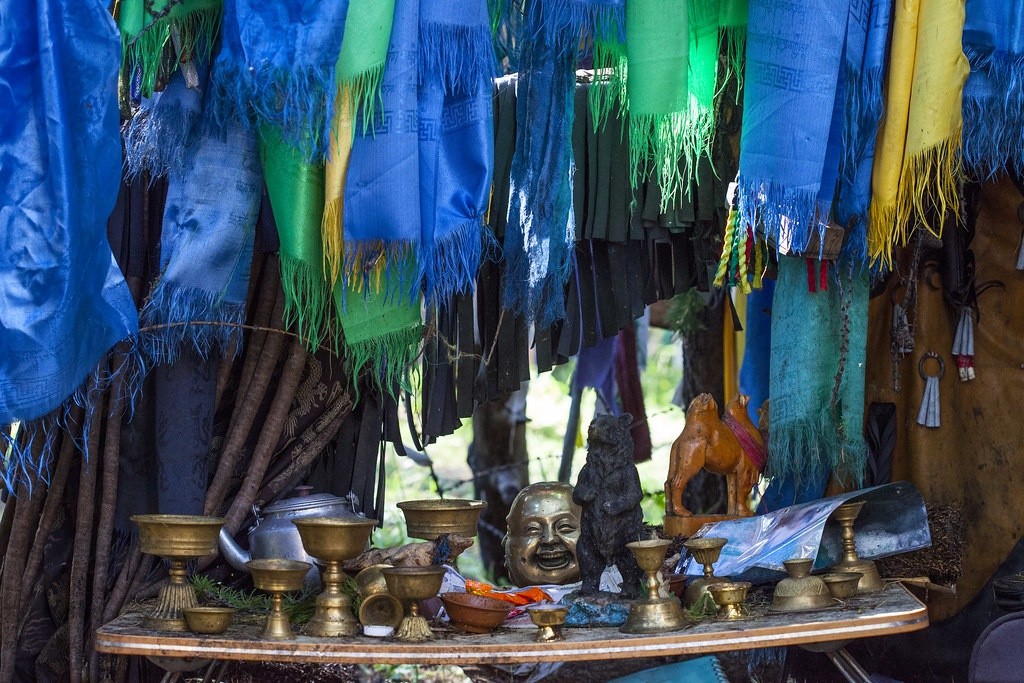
[217,486,365,588]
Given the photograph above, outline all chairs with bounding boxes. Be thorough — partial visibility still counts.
[968,611,1024,683]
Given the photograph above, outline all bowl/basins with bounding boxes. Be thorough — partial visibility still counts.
[821,572,863,598]
[439,592,513,635]
[663,574,688,599]
[182,607,237,634]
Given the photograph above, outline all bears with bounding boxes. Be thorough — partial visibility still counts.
[570,413,649,600]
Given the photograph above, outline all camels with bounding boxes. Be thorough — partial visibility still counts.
[665,393,765,513]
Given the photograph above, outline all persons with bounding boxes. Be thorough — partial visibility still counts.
[501,481,583,587]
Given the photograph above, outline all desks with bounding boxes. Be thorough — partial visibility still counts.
[96,579,930,683]
